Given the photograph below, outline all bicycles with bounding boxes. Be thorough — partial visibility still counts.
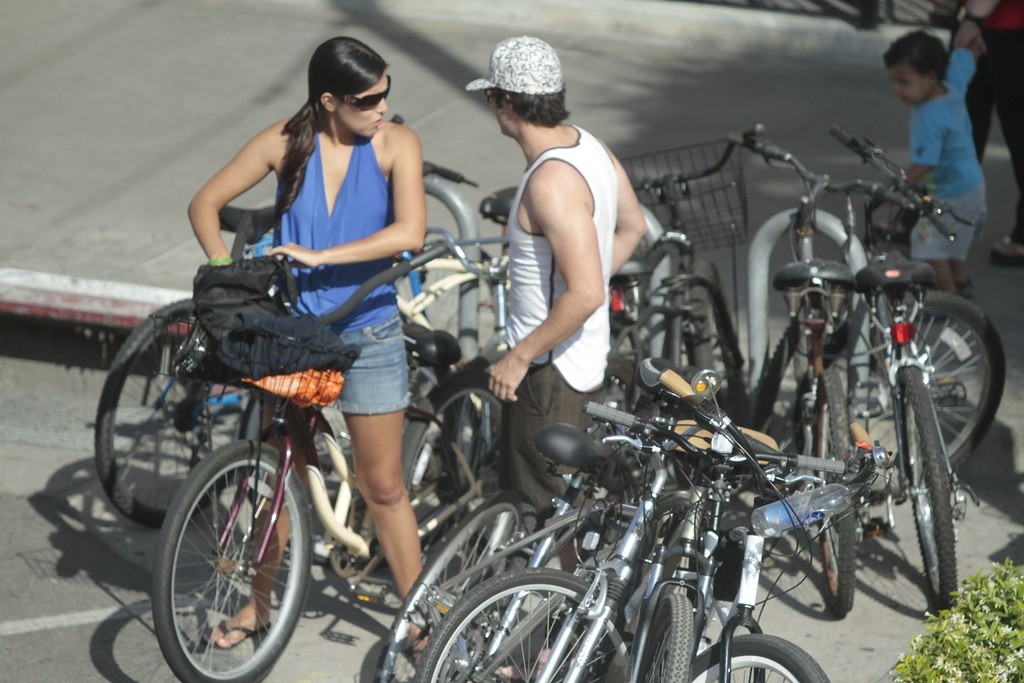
[94,123,1008,683]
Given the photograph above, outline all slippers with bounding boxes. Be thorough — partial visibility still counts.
[410,648,423,670]
[203,621,272,650]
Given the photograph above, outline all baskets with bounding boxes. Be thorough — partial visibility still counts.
[622,137,750,251]
[149,314,357,408]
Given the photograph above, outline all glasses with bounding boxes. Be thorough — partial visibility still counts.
[483,88,519,106]
[332,75,391,106]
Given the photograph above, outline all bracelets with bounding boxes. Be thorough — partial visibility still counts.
[207,257,233,267]
[965,13,983,27]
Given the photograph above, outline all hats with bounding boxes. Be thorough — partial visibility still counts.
[465,36,563,95]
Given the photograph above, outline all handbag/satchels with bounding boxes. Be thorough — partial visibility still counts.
[171,252,360,390]
[239,368,346,408]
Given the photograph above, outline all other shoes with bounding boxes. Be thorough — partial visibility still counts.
[991,237,1024,267]
[956,279,974,301]
[875,224,912,248]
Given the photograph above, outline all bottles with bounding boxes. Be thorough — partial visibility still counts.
[752,482,869,538]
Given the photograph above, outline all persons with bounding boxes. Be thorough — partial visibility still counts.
[949,0,1024,264]
[884,30,987,322]
[189,35,431,666]
[466,36,644,681]
